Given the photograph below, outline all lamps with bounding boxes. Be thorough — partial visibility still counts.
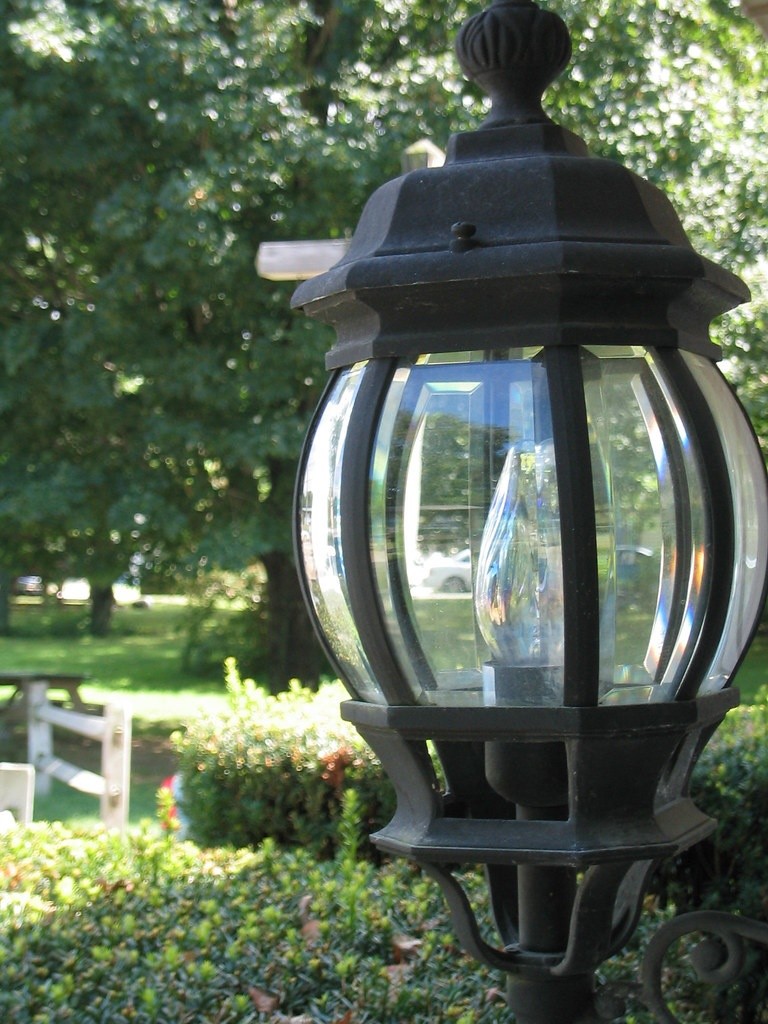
[292,0,768,1024]
